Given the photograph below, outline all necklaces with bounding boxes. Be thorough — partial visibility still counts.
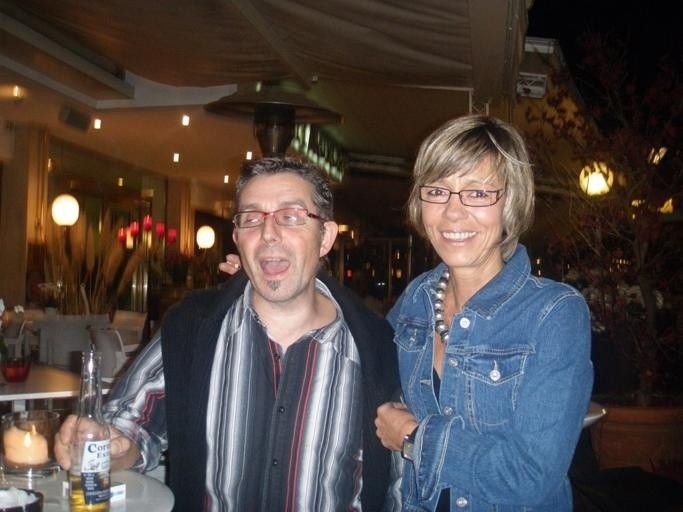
[431,266,452,346]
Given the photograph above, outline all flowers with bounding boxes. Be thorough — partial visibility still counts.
[511,78,679,400]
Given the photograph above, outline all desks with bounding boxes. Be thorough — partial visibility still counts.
[0,358,113,416]
[1,459,177,511]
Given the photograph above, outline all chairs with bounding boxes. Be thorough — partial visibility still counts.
[1,304,148,379]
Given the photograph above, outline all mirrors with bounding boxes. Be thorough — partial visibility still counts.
[55,179,153,315]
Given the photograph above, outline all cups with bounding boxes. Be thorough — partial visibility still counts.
[0,407,62,480]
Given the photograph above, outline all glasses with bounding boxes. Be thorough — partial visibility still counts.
[417,183,507,208]
[230,206,324,230]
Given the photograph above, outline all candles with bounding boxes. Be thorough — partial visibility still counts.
[113,216,178,246]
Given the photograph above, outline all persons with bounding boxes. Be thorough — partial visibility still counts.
[51,150,403,510]
[213,112,594,510]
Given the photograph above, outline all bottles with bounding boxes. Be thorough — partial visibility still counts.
[67,351,112,512]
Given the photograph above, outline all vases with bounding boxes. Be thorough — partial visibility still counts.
[587,394,682,477]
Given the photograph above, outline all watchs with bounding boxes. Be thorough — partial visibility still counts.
[400,426,419,463]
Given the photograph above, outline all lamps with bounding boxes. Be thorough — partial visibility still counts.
[197,71,344,165]
